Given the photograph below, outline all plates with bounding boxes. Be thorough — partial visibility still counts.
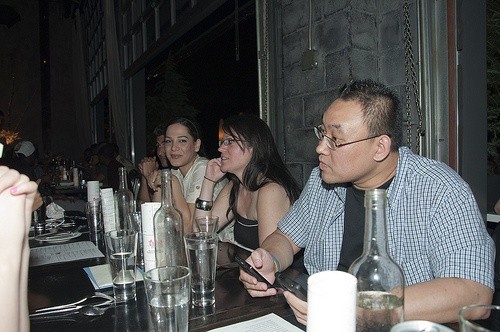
[35,231,82,243]
[390,320,455,332]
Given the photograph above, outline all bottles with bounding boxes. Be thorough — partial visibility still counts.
[348,189,405,332]
[154,168,190,281]
[114,167,136,233]
[49,152,83,181]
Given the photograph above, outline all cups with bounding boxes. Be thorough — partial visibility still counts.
[53,171,62,189]
[458,305,500,332]
[195,216,218,236]
[104,229,138,302]
[86,203,102,231]
[184,232,218,307]
[144,266,191,332]
[127,212,144,269]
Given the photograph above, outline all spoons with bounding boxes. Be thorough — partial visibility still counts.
[29,306,105,316]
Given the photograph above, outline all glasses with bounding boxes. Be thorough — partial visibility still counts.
[313,124,392,150]
[219,140,247,147]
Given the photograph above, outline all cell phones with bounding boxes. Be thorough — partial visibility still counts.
[274,272,307,302]
[234,255,278,292]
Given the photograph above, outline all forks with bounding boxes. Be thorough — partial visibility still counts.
[36,292,115,313]
[29,226,83,237]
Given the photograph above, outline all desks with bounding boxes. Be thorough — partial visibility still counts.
[29,184,308,332]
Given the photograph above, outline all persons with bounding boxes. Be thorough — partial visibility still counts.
[0,129,52,332]
[148,119,235,241]
[240,80,495,328]
[81,142,132,189]
[137,131,170,201]
[192,114,305,273]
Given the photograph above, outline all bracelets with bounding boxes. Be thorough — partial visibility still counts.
[204,176,215,183]
[272,256,280,273]
[195,198,212,210]
